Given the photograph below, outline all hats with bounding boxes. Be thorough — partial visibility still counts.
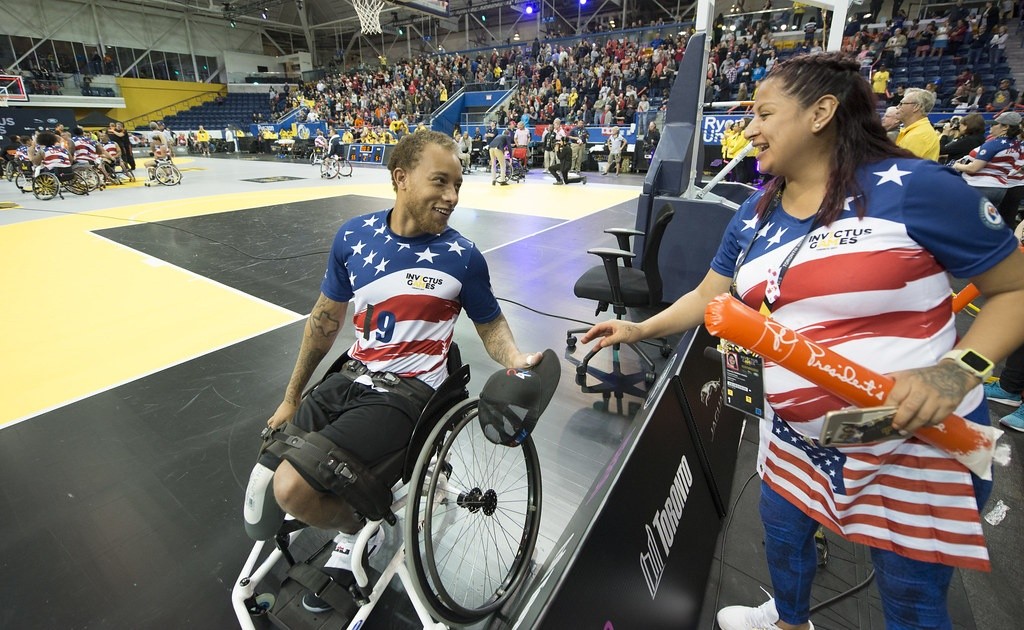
[985,113,1022,126]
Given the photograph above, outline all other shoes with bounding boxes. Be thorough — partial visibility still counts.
[582,176,587,185]
[602,172,608,176]
[492,180,496,185]
[554,180,562,185]
[814,532,826,566]
[501,182,509,185]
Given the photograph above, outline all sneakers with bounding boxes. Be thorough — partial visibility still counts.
[303,518,386,612]
[982,376,1022,408]
[999,402,1024,432]
[717,589,816,630]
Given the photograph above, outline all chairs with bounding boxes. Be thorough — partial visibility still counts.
[135,93,295,131]
[566,202,675,397]
[647,32,1019,106]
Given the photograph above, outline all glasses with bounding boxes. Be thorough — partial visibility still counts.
[898,100,921,108]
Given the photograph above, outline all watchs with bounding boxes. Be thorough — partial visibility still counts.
[940,349,995,385]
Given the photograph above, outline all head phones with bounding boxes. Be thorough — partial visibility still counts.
[563,136,567,145]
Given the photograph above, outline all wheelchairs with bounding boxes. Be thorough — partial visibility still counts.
[229,338,545,630]
[310,146,328,165]
[320,145,351,178]
[5,159,182,202]
[496,157,523,184]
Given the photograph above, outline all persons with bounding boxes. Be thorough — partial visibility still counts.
[582,51,1024,630]
[252,0,1024,431]
[0,50,235,192]
[244,130,543,612]
